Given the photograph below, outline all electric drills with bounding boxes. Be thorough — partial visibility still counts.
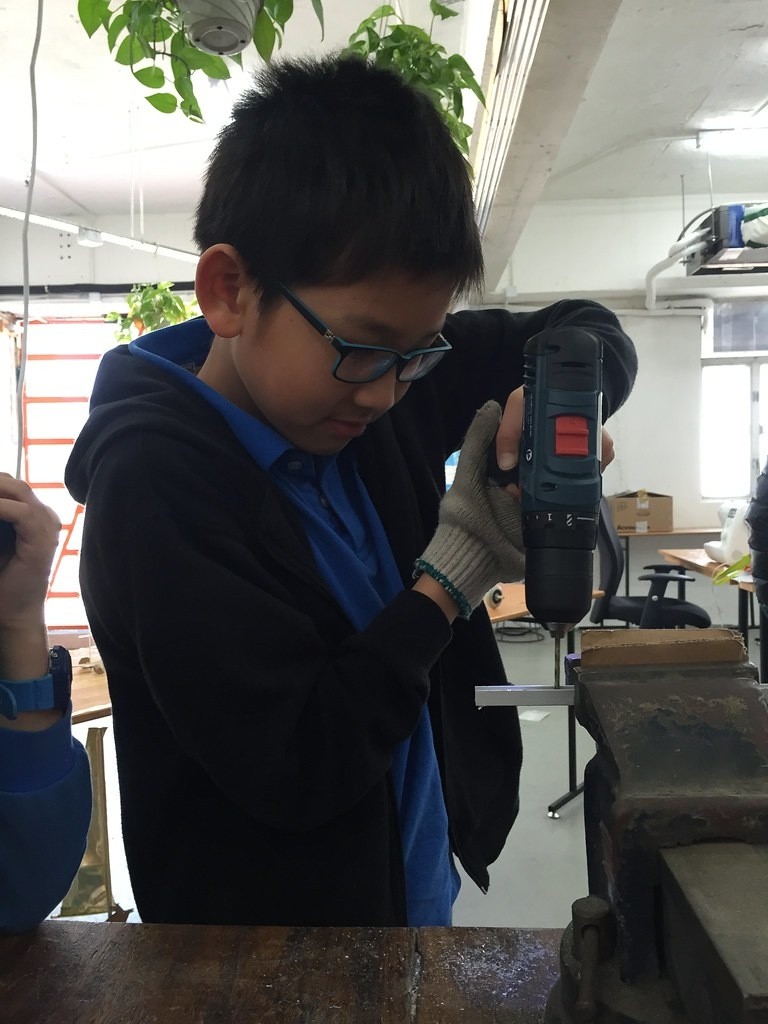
[482,328,604,687]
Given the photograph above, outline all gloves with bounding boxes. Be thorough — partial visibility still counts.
[417,399,526,616]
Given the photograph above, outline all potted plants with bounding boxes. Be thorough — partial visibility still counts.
[77,0,324,126]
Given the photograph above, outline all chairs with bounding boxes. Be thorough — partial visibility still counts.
[590,494,713,630]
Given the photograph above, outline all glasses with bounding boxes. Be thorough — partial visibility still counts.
[280,284,453,384]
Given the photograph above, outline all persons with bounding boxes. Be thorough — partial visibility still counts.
[2,55,638,934]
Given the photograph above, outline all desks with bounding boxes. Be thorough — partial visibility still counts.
[482,582,606,654]
[659,547,768,684]
[578,527,760,630]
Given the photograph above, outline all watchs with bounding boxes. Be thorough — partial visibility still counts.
[0,645,72,720]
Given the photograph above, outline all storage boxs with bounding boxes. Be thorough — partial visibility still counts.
[606,490,673,533]
